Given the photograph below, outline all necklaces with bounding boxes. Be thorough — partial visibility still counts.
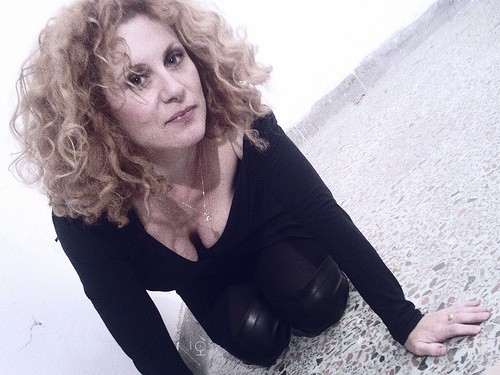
[139,149,212,222]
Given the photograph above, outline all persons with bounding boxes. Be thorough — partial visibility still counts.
[11,1,491,375]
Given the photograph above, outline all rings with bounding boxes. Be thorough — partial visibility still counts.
[448,313,454,324]
